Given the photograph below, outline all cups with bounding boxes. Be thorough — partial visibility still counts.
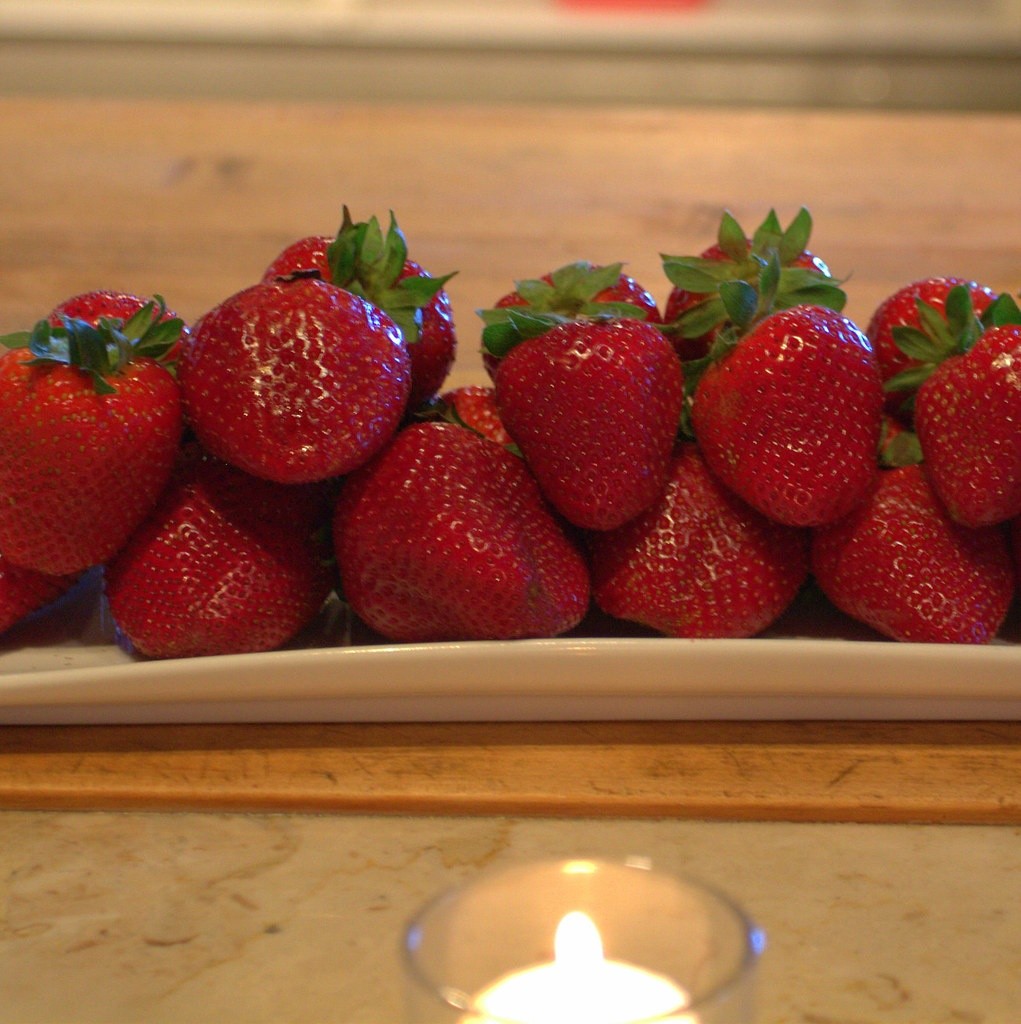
[400,847,767,1024]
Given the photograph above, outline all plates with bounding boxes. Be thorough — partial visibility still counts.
[3,572,1021,720]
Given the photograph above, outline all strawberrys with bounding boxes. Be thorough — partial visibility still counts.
[0,200,1020,689]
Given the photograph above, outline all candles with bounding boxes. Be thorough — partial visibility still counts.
[469,919,707,1024]
